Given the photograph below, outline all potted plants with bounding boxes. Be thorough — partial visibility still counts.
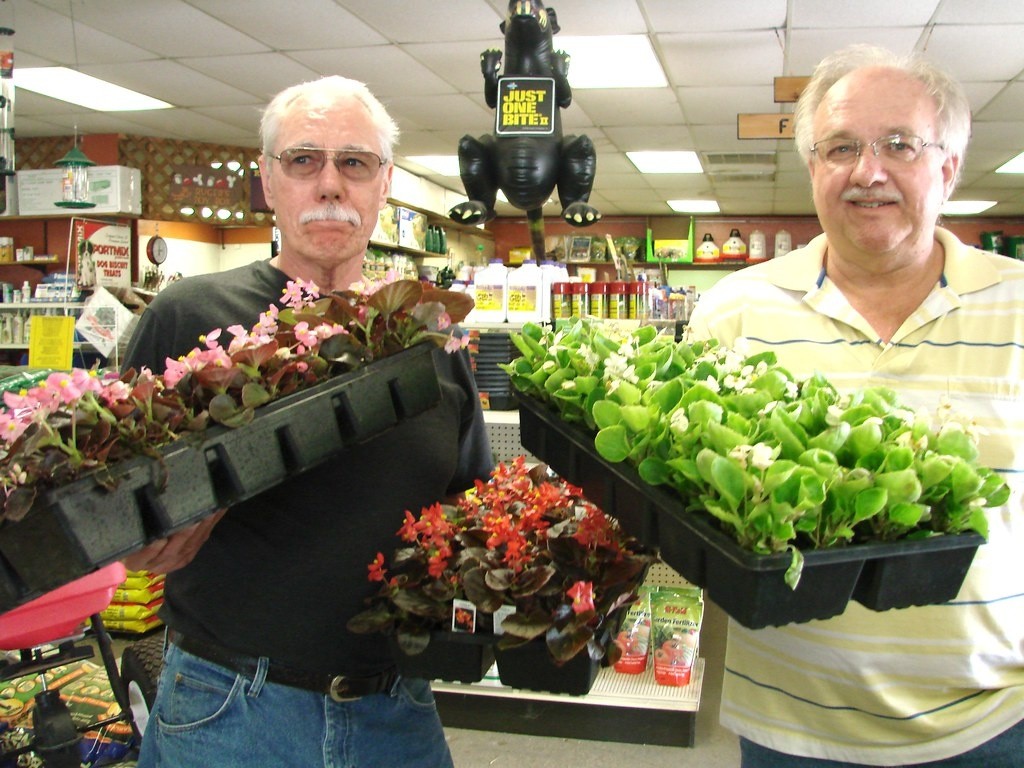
[497,318,1009,631]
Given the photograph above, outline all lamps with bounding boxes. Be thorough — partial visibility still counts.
[51,123,95,209]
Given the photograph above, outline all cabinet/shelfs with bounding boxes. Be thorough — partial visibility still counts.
[0,214,140,351]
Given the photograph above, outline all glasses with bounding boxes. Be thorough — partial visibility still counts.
[809,133,944,163]
[269,147,388,181]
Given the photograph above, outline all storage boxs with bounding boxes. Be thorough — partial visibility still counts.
[6,165,143,214]
[371,203,427,253]
[646,215,693,263]
[509,247,532,263]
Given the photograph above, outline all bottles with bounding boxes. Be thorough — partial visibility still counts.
[695,228,792,263]
[0,271,87,345]
[426,224,447,255]
[437,259,697,333]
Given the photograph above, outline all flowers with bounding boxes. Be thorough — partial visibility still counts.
[0,267,480,523]
[346,456,660,670]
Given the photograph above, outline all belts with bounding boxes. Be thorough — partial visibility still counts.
[168,628,391,701]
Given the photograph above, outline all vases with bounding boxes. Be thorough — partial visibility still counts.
[496,640,602,697]
[399,630,496,683]
[0,340,444,616]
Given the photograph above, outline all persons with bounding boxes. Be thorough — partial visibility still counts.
[683,44,1024,768]
[120,75,494,768]
